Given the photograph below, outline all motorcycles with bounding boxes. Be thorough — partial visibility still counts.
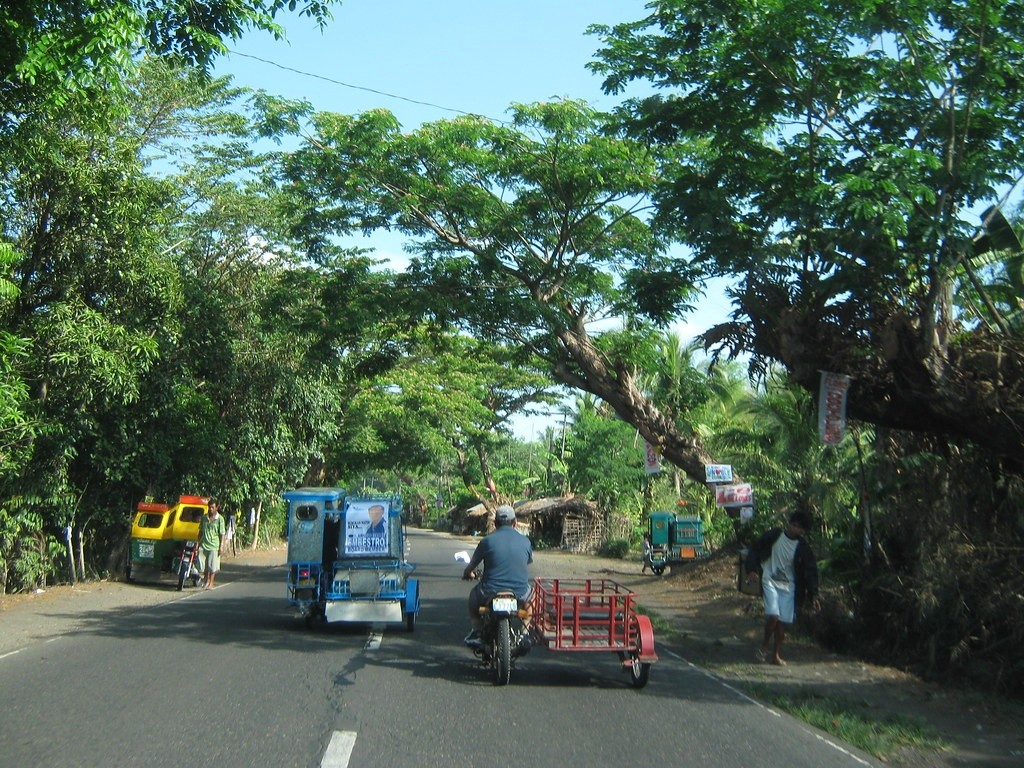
[648,511,703,577]
[455,550,660,687]
[282,486,419,634]
[131,494,210,589]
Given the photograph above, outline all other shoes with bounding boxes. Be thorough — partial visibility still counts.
[756,649,766,661]
[773,656,787,666]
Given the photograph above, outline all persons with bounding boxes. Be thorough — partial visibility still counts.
[196,500,225,590]
[641,532,651,574]
[366,505,387,533]
[464,505,536,646]
[745,511,821,666]
[441,514,452,532]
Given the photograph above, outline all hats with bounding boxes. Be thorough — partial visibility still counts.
[496,505,515,520]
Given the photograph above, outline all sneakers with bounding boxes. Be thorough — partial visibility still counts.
[464,629,481,646]
[517,634,532,646]
